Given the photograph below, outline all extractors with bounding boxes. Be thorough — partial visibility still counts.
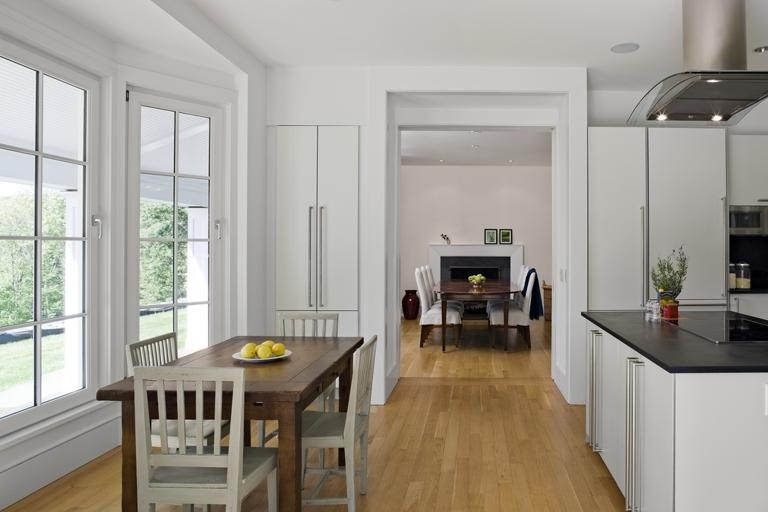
[648,69,768,123]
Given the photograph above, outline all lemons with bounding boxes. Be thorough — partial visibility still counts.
[240,342,257,358]
[262,339,275,349]
[272,342,286,356]
[255,344,266,354]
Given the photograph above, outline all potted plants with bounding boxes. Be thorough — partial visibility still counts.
[469,274,486,287]
[650,248,686,325]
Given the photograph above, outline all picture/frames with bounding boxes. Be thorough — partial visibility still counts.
[483,228,498,245]
[499,227,513,245]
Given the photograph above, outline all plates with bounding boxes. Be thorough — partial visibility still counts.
[230,344,293,365]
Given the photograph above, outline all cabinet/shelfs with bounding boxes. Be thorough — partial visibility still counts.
[265,124,361,312]
[615,341,675,512]
[582,317,614,464]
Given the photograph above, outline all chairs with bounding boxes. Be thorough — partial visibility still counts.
[287,335,379,512]
[258,311,338,469]
[132,366,280,512]
[124,331,251,511]
[490,265,537,349]
[416,265,463,348]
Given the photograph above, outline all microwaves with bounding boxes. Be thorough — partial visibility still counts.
[728,203,768,236]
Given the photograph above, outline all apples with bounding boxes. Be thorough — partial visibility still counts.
[255,345,271,359]
[468,276,473,281]
[472,276,481,284]
[480,276,486,283]
[476,273,482,280]
[471,275,476,280]
